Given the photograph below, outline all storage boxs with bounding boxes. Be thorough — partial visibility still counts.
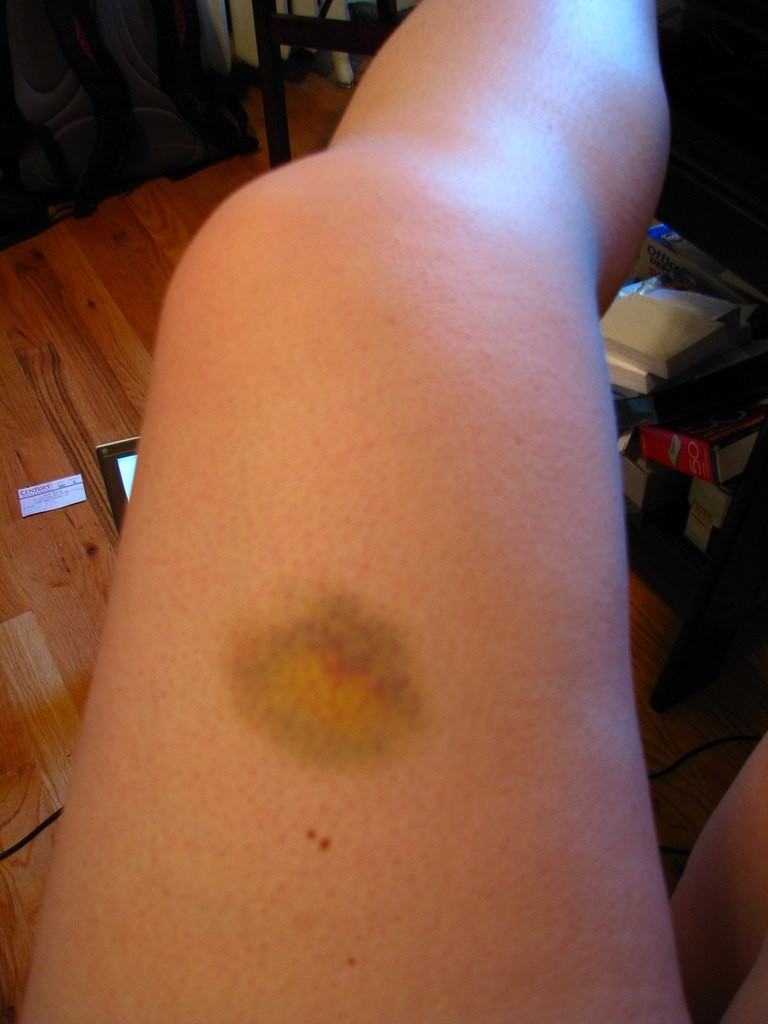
[600,222,768,553]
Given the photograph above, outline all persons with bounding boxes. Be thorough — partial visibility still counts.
[13,1,767,1024]
